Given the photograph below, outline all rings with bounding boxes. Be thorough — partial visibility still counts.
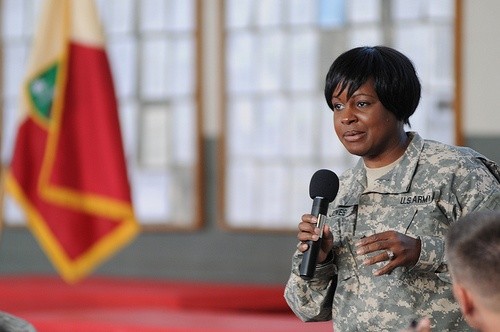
[385,250,393,259]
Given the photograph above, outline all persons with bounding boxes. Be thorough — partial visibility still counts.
[398,210,500,332]
[284,46,500,332]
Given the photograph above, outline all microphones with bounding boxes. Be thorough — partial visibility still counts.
[299,169,339,280]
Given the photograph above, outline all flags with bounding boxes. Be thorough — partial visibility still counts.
[6,0,139,283]
